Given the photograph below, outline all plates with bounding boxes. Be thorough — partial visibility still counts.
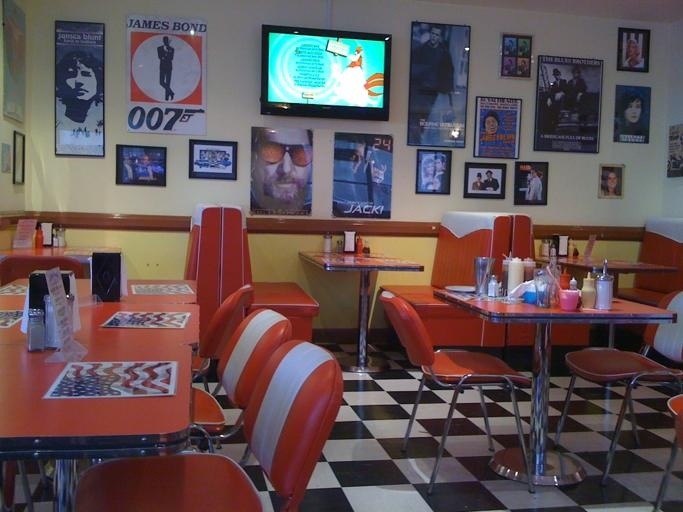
[444,285,477,293]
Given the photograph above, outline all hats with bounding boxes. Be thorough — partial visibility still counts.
[476,171,492,177]
[552,68,560,76]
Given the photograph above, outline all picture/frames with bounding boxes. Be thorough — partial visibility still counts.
[500,34,533,79]
[473,96,522,159]
[53,20,106,158]
[615,26,651,74]
[11,130,26,187]
[188,137,238,182]
[597,163,626,199]
[115,144,167,186]
[534,54,604,154]
[462,161,507,200]
[415,148,453,195]
[406,20,471,148]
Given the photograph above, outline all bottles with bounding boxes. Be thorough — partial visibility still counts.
[323,231,371,256]
[32,220,66,248]
[24,308,45,352]
[540,234,580,258]
[42,291,77,350]
[472,250,616,312]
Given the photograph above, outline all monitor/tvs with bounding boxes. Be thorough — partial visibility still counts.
[261,25,392,120]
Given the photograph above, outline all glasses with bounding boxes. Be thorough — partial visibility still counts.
[252,141,313,168]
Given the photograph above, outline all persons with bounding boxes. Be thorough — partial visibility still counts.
[335,135,363,211]
[504,36,529,75]
[624,39,644,69]
[157,36,175,101]
[422,154,445,191]
[614,91,647,142]
[123,154,155,180]
[603,169,618,196]
[526,167,542,200]
[408,25,454,142]
[472,171,499,190]
[562,67,586,123]
[546,68,566,125]
[55,48,104,135]
[483,110,501,137]
[252,126,312,213]
